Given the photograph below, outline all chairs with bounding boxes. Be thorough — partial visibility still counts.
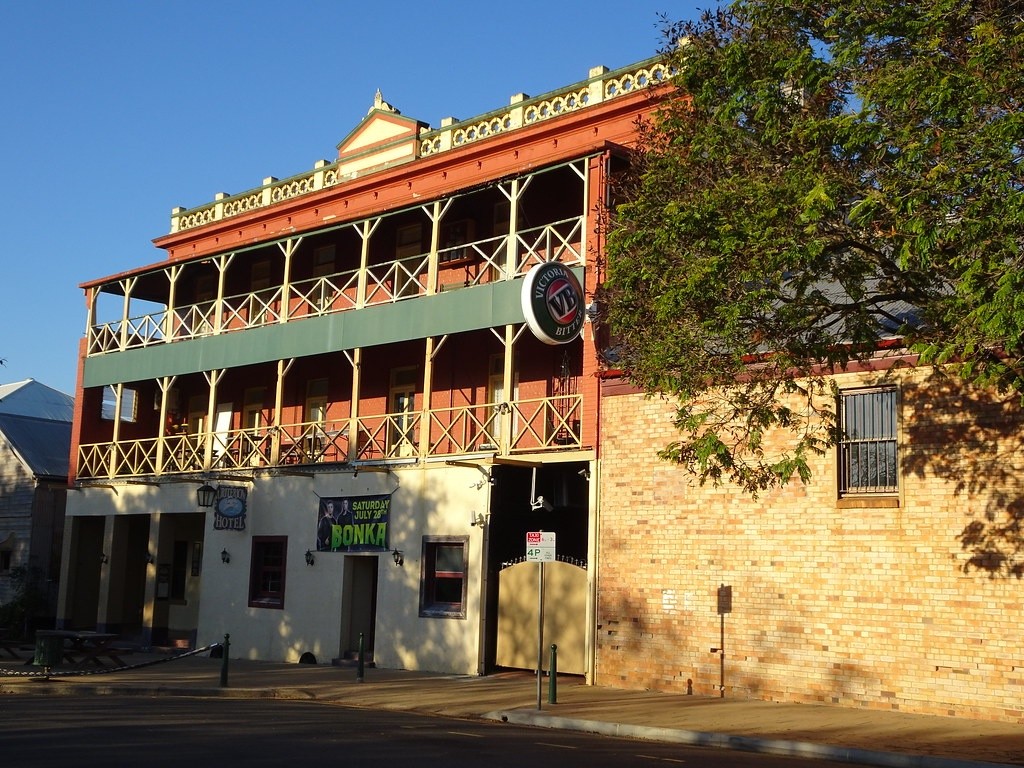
[319,424,338,462]
[548,417,580,444]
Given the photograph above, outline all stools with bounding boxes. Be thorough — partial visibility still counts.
[228,448,247,465]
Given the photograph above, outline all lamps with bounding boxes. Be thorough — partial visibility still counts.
[221,548,230,563]
[99,552,108,564]
[145,549,153,565]
[196,482,222,509]
[304,549,314,567]
[560,350,571,378]
[392,548,403,567]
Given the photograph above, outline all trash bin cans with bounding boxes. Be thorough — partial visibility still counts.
[32,629,63,668]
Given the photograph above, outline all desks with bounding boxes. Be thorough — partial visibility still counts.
[177,449,219,470]
[0,628,21,659]
[226,436,272,466]
[24,630,128,667]
[285,427,372,462]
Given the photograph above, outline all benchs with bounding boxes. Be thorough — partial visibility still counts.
[0,640,25,647]
[20,644,134,658]
